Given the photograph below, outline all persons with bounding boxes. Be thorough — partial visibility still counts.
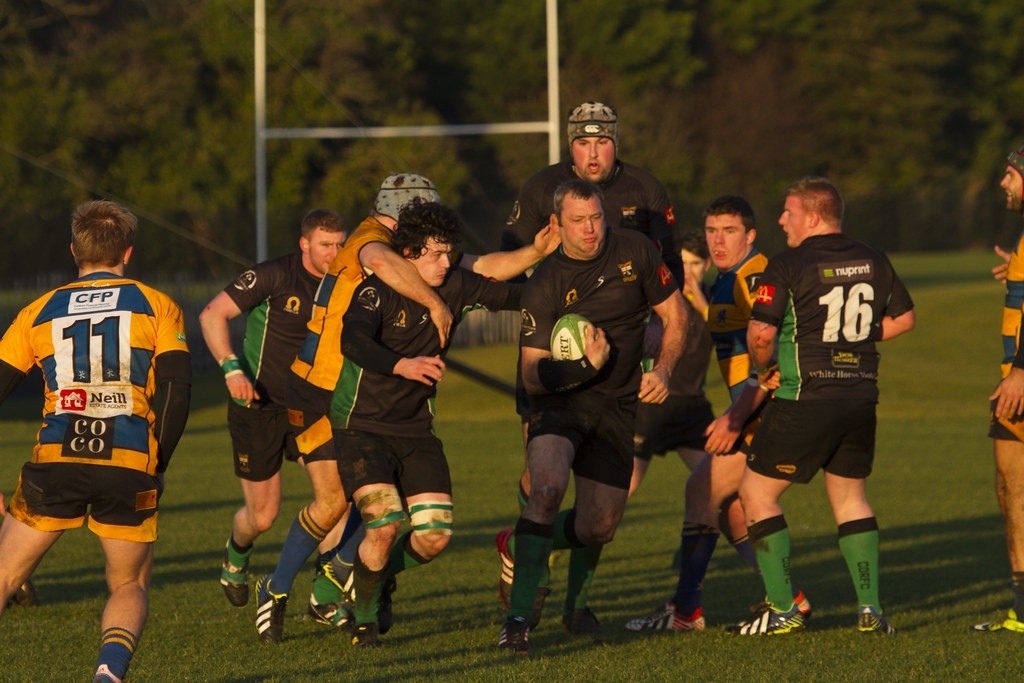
[0,494,39,608]
[199,99,917,654]
[0,199,193,683]
[971,146,1024,635]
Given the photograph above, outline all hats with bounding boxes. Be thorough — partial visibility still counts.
[375,174,440,222]
[567,101,619,161]
[1008,147,1024,178]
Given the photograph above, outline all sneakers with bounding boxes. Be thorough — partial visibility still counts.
[352,624,381,648]
[969,605,1024,633]
[255,574,289,647]
[562,606,602,636]
[307,591,355,631]
[857,607,897,635]
[498,616,531,656]
[496,528,550,629]
[724,591,813,637]
[220,536,249,607]
[317,549,357,602]
[625,600,705,631]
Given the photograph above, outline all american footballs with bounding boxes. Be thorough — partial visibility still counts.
[550,314,598,361]
